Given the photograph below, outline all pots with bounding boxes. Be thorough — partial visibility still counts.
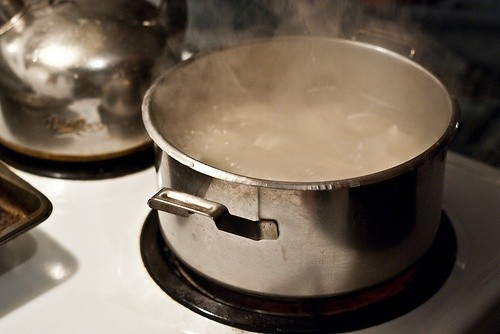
[142,23,458,317]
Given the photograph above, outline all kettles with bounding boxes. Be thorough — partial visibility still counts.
[0,0,191,161]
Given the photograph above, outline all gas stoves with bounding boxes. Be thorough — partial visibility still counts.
[1,153,500,334]
